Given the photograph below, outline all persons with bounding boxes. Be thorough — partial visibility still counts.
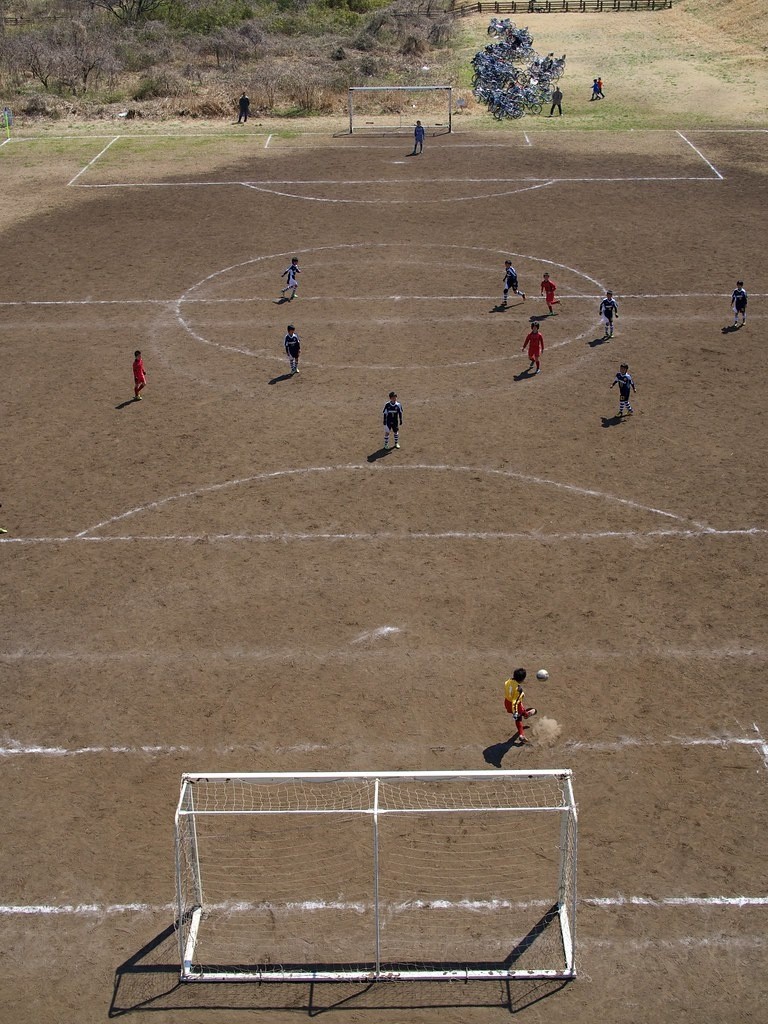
[599,291,618,337]
[549,87,563,117]
[281,257,301,298]
[133,351,147,399]
[237,92,249,123]
[589,77,605,100]
[500,260,526,306]
[381,392,403,449]
[522,321,544,373]
[730,281,747,327]
[540,273,562,316]
[284,325,301,372]
[503,667,537,743]
[609,365,636,417]
[411,121,424,154]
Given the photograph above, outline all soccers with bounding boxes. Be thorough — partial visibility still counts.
[536,669,549,682]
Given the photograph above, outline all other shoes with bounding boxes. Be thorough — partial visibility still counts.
[530,361,534,366]
[524,708,535,719]
[501,302,507,306]
[519,735,528,743]
[616,411,623,417]
[557,299,562,306]
[734,323,737,327]
[627,410,633,415]
[281,290,284,296]
[605,332,614,338]
[395,443,400,449]
[536,369,540,374]
[291,294,298,298]
[742,321,746,326]
[384,445,389,449]
[522,293,527,300]
[291,368,300,374]
[135,391,142,400]
[548,312,556,316]
